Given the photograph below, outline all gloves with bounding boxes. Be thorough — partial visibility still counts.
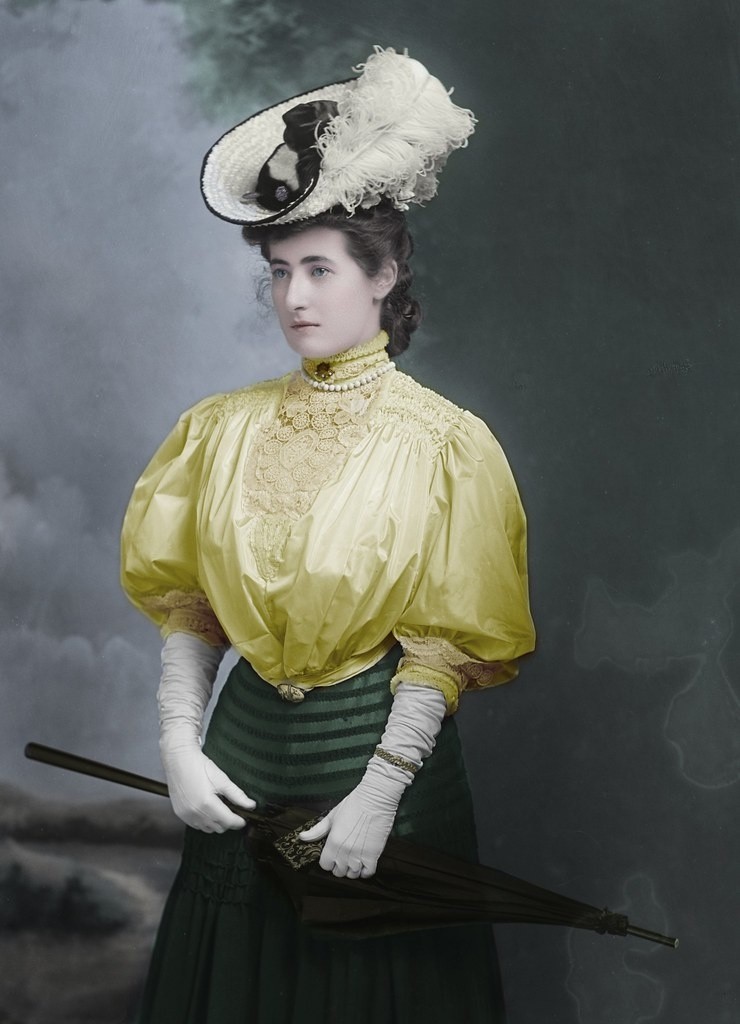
[156,631,256,835]
[298,684,446,880]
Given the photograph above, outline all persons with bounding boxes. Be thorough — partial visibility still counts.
[120,55,537,1024]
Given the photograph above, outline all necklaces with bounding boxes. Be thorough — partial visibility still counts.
[303,362,396,393]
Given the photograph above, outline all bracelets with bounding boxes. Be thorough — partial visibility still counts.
[375,748,416,773]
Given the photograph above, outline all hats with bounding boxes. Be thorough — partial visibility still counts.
[199,45,480,229]
